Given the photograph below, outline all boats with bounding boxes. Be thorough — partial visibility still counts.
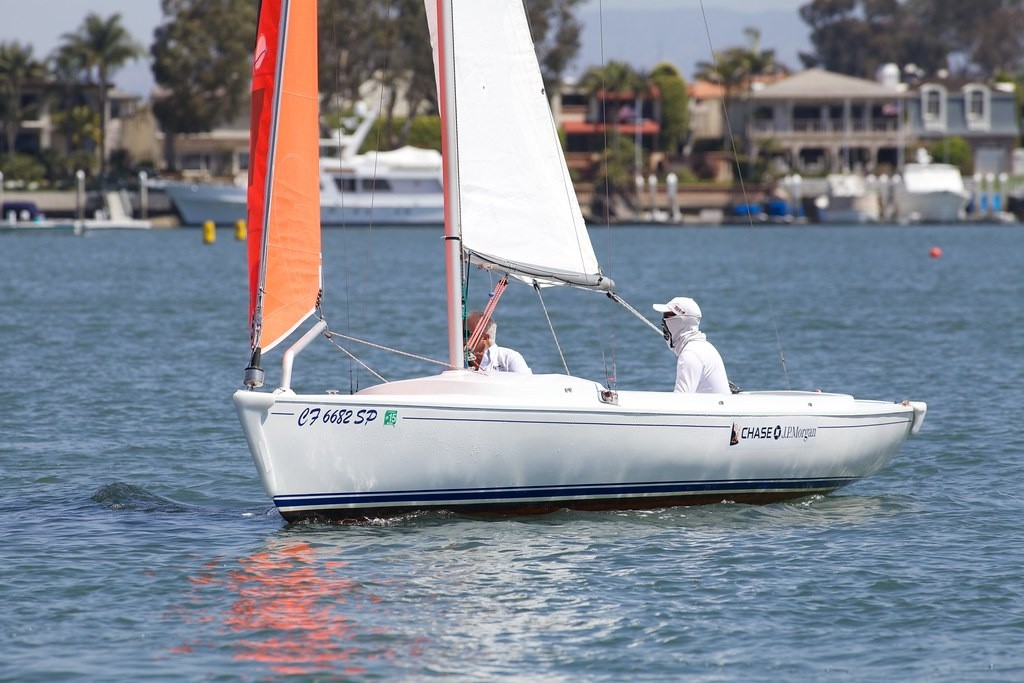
[159,105,450,232]
[895,159,971,227]
[811,170,880,223]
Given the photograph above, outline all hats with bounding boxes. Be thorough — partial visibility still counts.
[653,297,702,318]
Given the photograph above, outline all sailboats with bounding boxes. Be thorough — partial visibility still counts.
[233,1,928,527]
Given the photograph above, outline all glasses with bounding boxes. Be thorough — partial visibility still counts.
[662,314,671,319]
[463,330,473,338]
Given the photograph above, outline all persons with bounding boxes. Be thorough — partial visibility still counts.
[462,312,532,372]
[651,297,732,393]
